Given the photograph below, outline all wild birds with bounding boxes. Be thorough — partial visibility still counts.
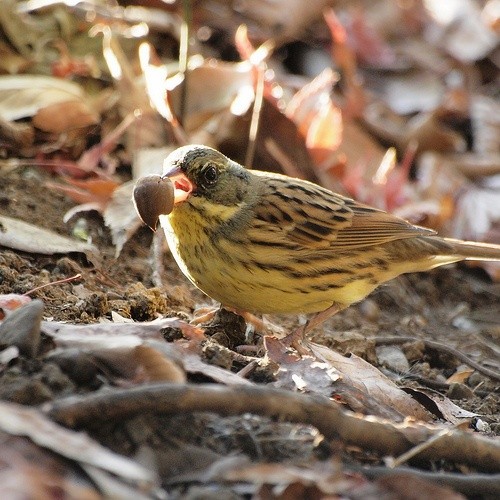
[157,142,499,359]
